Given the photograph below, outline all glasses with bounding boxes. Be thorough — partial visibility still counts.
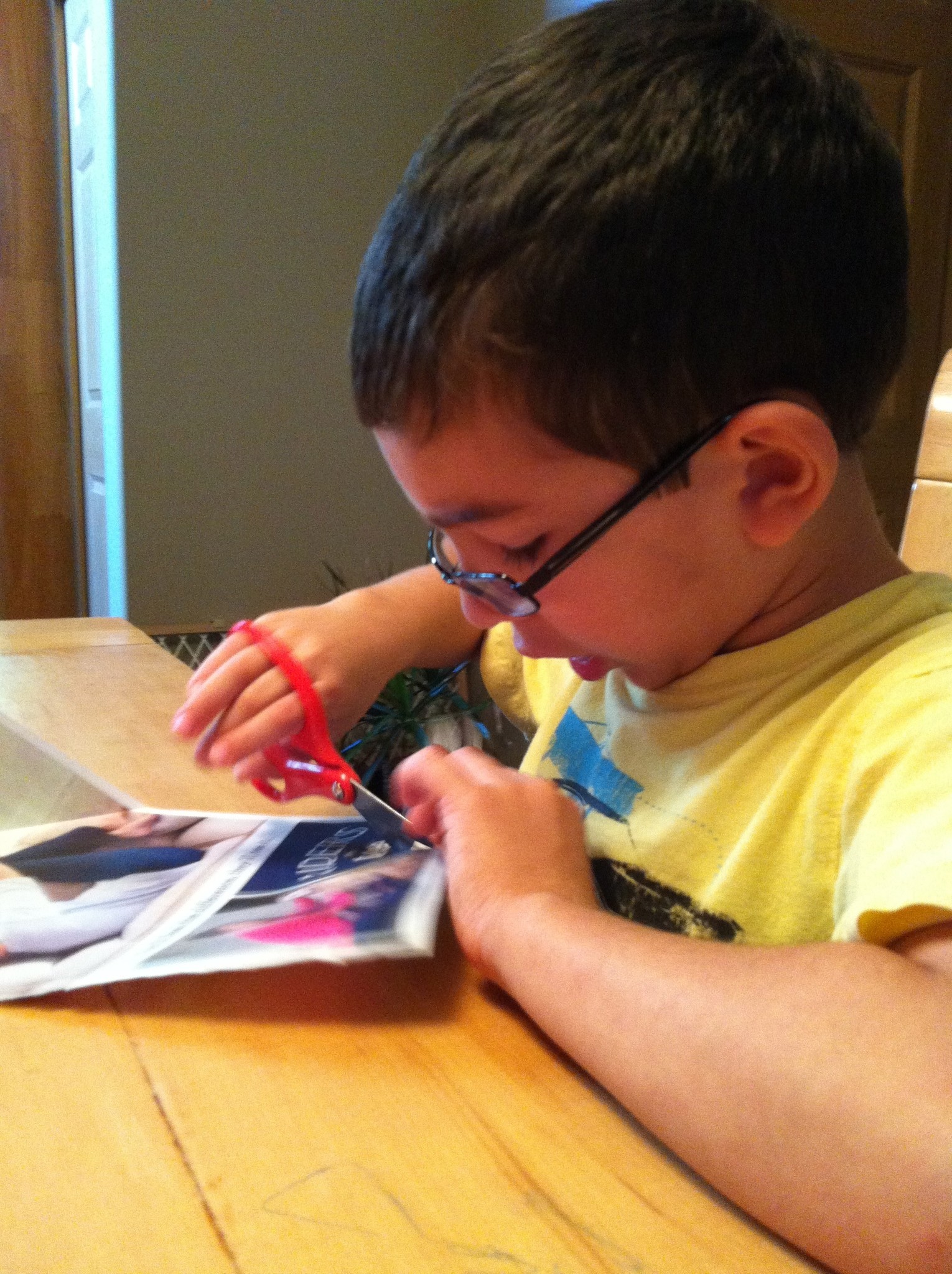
[425,388,827,618]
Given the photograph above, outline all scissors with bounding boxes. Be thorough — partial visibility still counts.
[227,618,439,854]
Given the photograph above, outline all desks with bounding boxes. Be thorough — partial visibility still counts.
[1,613,839,1274]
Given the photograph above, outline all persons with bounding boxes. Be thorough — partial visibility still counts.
[166,0,952,1274]
